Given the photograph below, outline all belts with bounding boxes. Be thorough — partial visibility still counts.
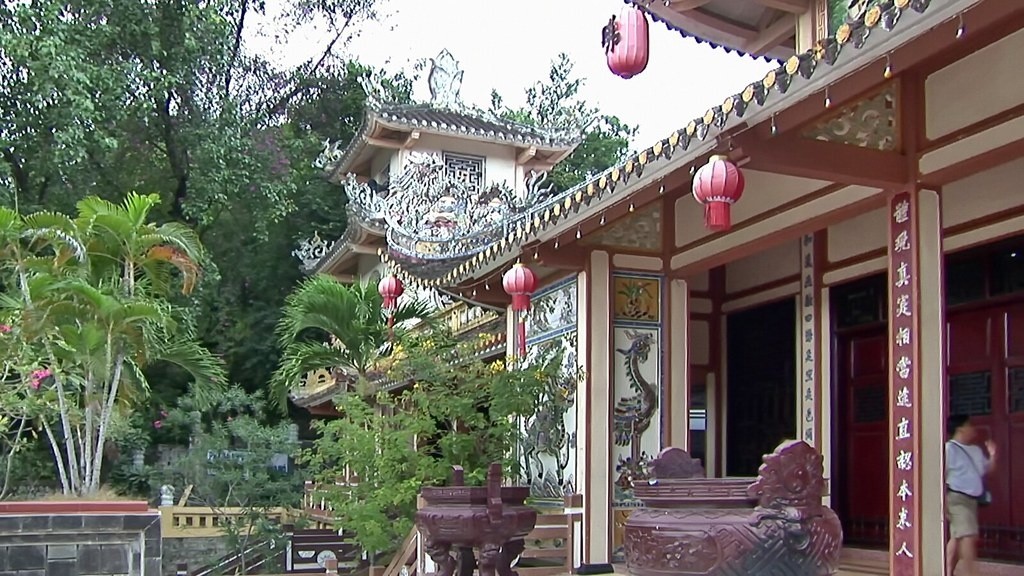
[948,487,981,499]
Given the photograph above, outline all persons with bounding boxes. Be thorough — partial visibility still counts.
[944,415,999,576]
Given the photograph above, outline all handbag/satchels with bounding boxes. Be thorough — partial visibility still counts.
[978,490,992,505]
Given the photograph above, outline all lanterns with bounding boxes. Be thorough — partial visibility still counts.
[692,155,744,233]
[379,275,403,327]
[602,4,650,78]
[503,262,537,355]
